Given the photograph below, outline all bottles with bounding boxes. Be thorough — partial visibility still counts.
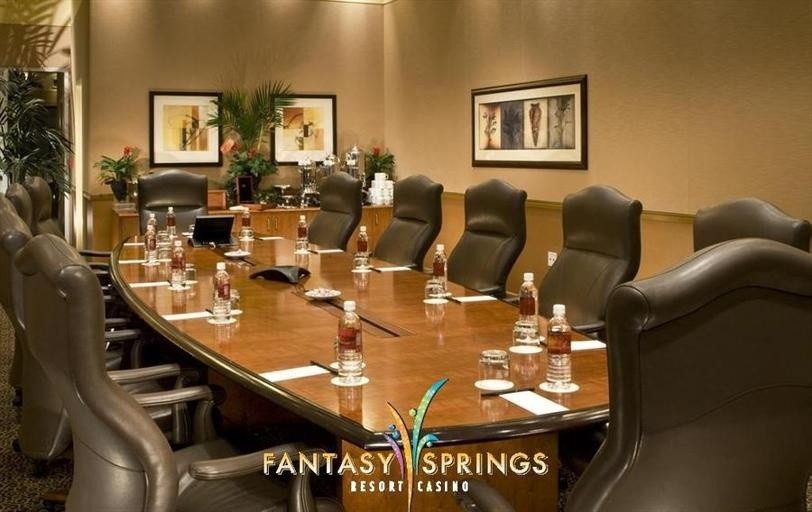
[237,208,370,272]
[518,272,539,321]
[335,301,364,373]
[338,387,363,424]
[544,304,573,391]
[431,242,447,293]
[300,142,364,192]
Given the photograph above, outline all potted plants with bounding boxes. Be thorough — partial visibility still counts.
[0,69,58,230]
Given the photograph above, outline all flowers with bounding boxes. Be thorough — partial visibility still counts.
[94,146,139,177]
[208,74,288,192]
[367,146,394,173]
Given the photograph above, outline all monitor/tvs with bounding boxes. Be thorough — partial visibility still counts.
[193,214,236,239]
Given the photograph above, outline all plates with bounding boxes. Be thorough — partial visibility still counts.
[304,288,342,301]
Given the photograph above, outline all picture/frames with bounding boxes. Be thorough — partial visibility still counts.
[149,90,337,166]
[471,75,587,167]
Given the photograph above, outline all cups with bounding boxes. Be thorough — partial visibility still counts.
[424,299,445,323]
[424,280,447,307]
[478,394,508,424]
[511,320,541,355]
[337,351,363,384]
[353,270,369,290]
[144,205,241,325]
[511,354,540,390]
[477,349,511,390]
[368,171,395,207]
